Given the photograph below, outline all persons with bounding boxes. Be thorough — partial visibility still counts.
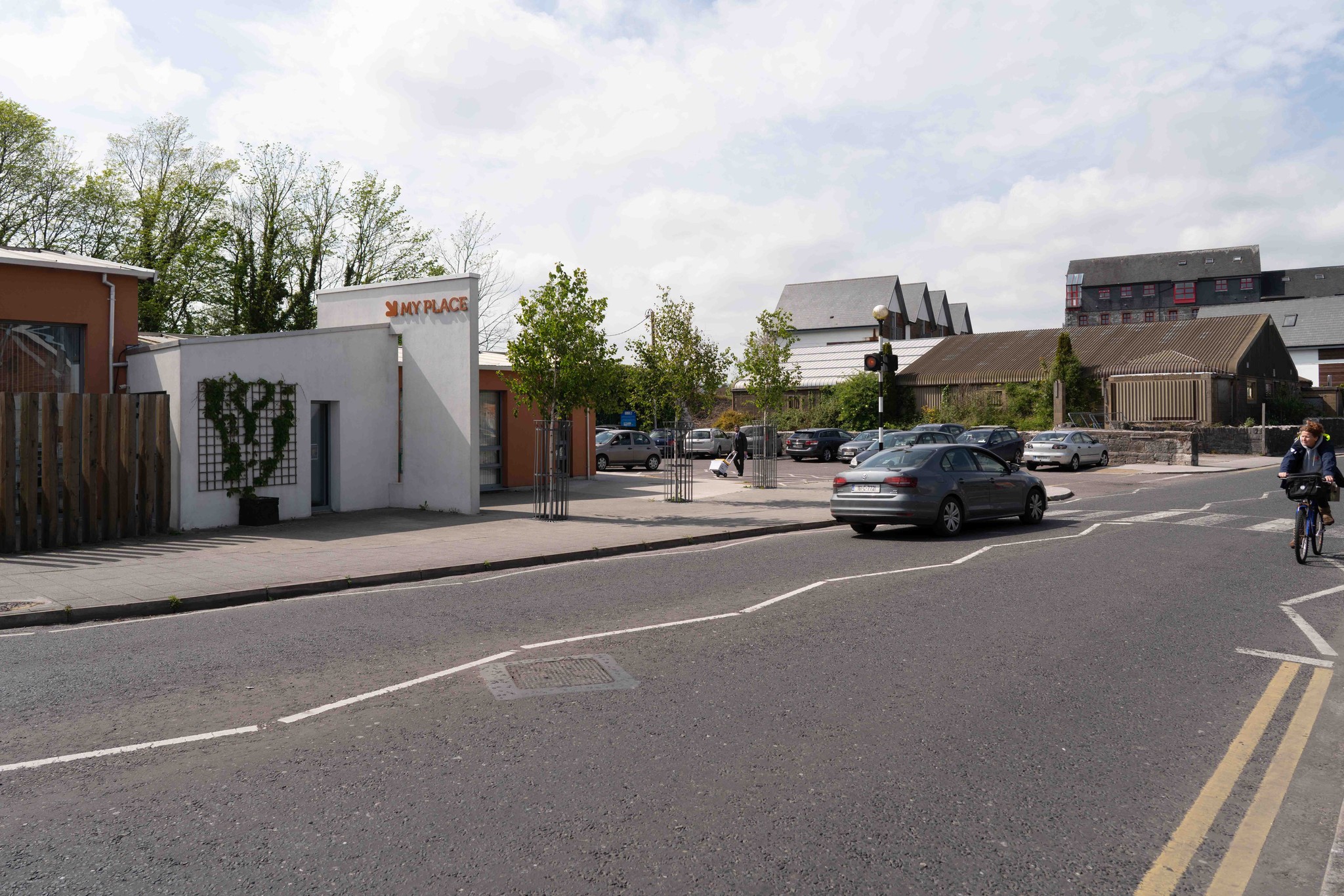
[1278,418,1344,547]
[732,424,748,476]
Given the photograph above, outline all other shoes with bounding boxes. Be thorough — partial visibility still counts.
[737,470,740,476]
[738,473,743,476]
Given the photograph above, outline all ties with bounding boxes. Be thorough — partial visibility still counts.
[736,434,738,439]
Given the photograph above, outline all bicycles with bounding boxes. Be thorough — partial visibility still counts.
[1277,473,1332,565]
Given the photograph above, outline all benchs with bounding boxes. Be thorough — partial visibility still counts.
[984,433,1007,441]
[919,439,948,443]
[951,429,958,435]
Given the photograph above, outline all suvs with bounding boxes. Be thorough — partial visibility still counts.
[739,424,783,457]
[909,423,967,440]
[683,427,733,459]
[955,425,1025,464]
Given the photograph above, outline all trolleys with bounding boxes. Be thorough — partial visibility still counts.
[715,451,737,478]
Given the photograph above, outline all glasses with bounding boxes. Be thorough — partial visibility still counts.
[734,428,739,430]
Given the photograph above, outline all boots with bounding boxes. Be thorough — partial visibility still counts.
[1289,528,1304,546]
[1318,505,1334,524]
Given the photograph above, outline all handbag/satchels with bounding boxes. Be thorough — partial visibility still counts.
[1286,472,1322,499]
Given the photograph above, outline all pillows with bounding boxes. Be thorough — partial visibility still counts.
[1049,438,1060,441]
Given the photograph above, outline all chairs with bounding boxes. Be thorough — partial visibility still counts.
[613,436,620,441]
[698,433,702,437]
[929,436,938,443]
[624,435,630,445]
[948,452,972,471]
[914,459,923,464]
[1041,437,1048,441]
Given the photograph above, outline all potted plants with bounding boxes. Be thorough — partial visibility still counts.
[202,371,295,526]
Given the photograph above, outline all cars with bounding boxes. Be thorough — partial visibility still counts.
[830,443,1048,537]
[557,423,662,471]
[785,427,855,463]
[645,427,687,457]
[849,430,958,470]
[837,428,904,463]
[1023,430,1109,472]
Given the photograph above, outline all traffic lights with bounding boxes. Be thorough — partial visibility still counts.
[864,354,881,371]
[883,354,898,372]
